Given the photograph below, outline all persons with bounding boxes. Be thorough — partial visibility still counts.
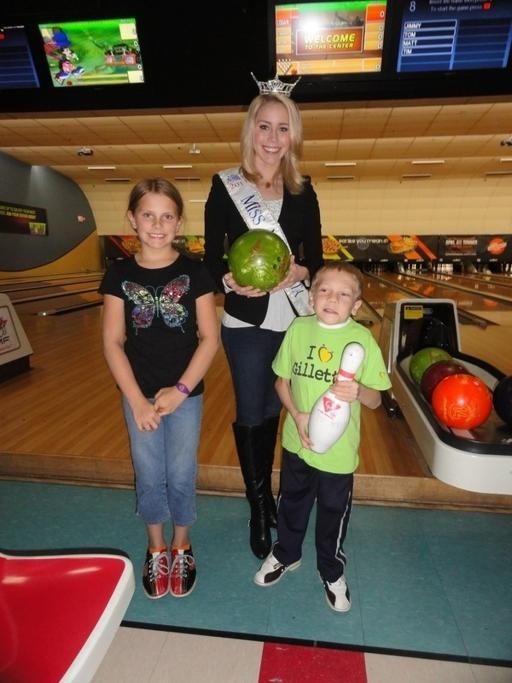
[253,261,391,612]
[203,92,324,561]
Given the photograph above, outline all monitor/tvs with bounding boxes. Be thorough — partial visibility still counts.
[0,15,145,90]
[274,0,512,77]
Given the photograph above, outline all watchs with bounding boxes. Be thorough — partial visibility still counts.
[176,382,190,395]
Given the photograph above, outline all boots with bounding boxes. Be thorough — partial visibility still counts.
[232,421,277,560]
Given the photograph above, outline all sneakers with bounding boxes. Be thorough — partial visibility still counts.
[142,545,171,600]
[252,549,304,587]
[168,544,197,598]
[317,569,352,612]
